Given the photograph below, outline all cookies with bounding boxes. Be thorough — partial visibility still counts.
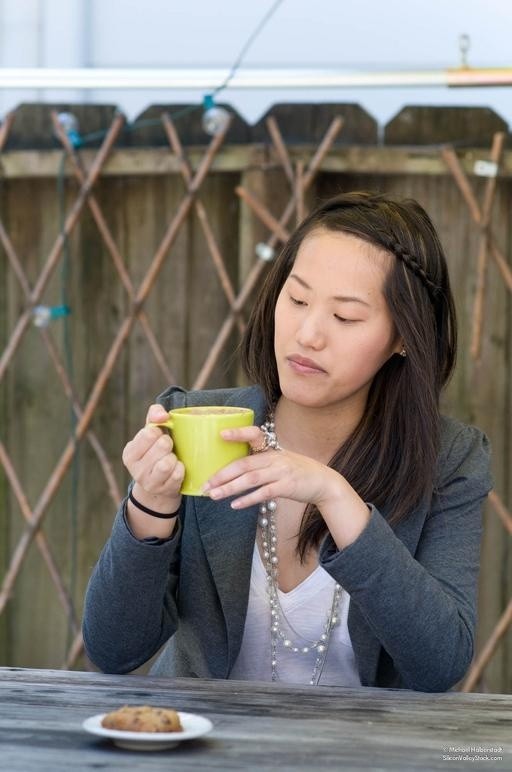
[104,705,182,732]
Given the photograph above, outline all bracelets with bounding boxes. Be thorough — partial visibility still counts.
[129,487,184,519]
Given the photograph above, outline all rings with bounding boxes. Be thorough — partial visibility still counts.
[252,425,282,452]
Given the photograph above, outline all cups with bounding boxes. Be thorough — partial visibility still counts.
[146,405,256,499]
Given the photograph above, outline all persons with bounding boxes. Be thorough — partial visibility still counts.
[81,191,496,692]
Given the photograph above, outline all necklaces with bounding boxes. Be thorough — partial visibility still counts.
[258,421,343,685]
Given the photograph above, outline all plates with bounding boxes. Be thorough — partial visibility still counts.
[82,708,213,752]
